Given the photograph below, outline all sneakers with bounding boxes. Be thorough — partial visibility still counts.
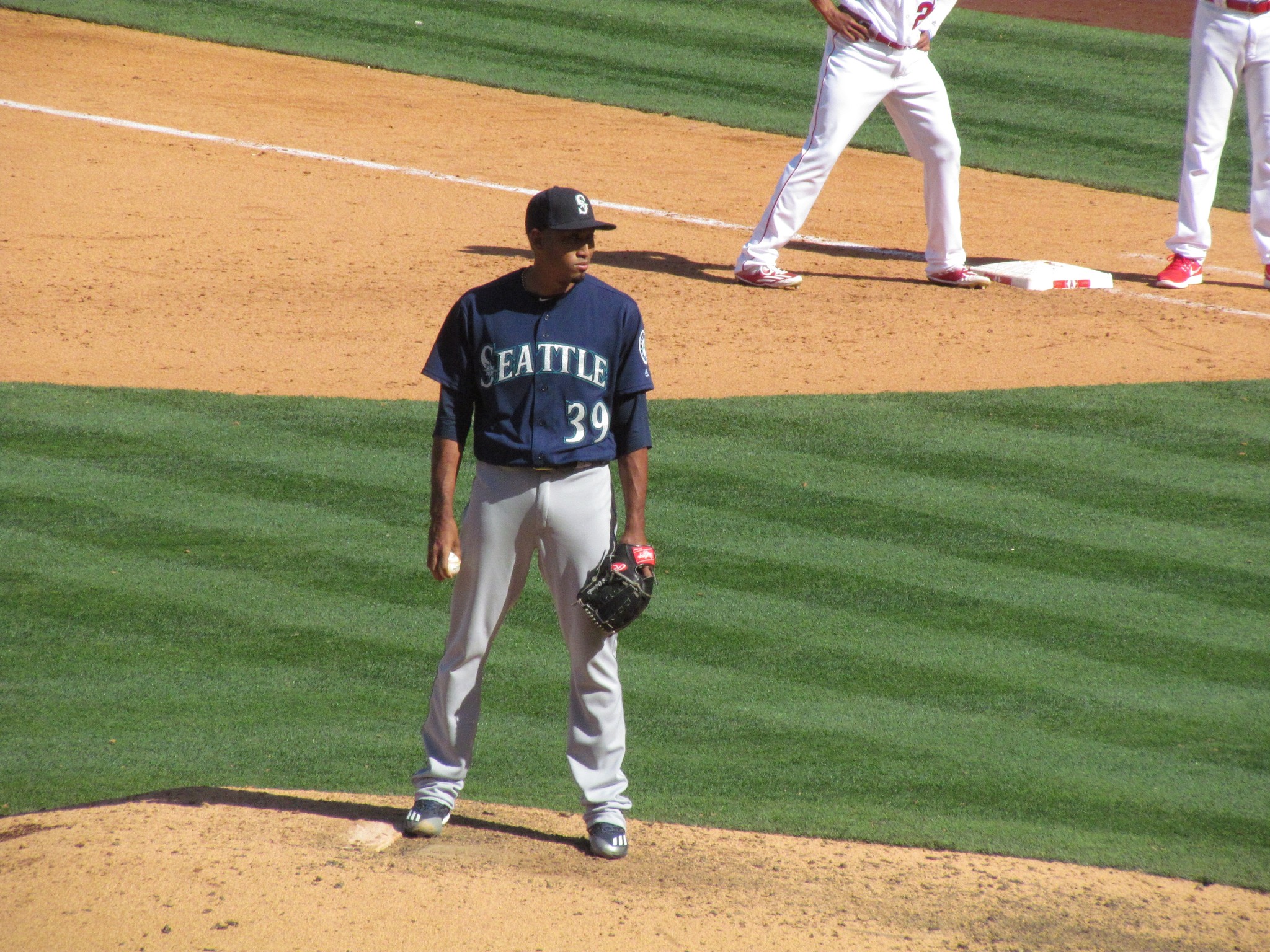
[1157,253,1203,288]
[588,822,628,860]
[1264,264,1270,289]
[735,266,802,289]
[405,800,450,836]
[928,268,992,290]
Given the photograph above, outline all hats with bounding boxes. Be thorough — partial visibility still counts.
[525,186,618,234]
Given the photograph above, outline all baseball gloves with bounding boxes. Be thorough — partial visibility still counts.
[576,544,656,637]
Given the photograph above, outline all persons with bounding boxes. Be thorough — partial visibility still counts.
[734,0,991,291]
[402,186,657,860]
[1156,0,1270,289]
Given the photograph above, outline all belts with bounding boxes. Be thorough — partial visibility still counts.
[533,460,577,470]
[1208,0,1270,13]
[863,23,906,50]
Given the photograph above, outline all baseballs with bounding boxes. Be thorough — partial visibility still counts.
[438,553,460,576]
